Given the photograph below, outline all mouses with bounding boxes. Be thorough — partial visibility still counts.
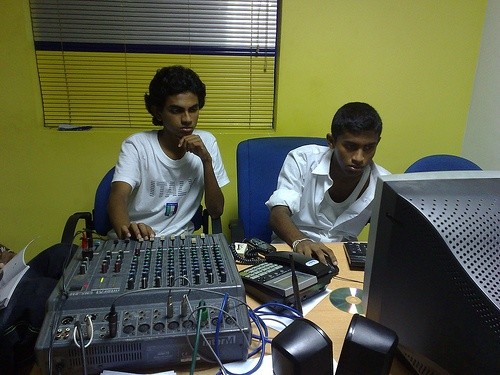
[311,253,339,275]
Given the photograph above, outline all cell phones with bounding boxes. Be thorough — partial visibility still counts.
[248,238,276,256]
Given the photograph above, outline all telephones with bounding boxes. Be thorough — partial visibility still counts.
[238,250,334,310]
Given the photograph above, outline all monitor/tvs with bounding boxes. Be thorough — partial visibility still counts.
[361,170,500,375]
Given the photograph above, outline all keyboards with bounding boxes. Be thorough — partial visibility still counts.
[343,242,368,267]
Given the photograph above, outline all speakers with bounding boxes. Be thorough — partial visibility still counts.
[270,316,333,375]
[335,314,398,375]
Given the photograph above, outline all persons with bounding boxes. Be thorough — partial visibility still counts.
[264,103,393,266]
[107,65,231,240]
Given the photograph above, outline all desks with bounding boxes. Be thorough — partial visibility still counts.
[30,241,365,375]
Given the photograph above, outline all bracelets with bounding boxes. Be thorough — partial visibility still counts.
[293,238,316,252]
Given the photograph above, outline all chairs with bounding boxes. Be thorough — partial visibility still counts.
[228,137,328,243]
[62,166,222,243]
